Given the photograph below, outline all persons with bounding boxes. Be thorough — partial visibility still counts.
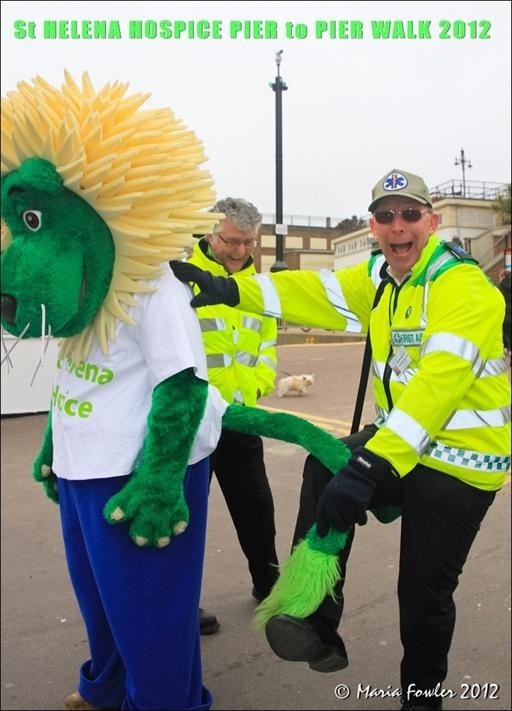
[496,268,512,350]
[166,167,511,710]
[0,69,359,710]
[183,197,283,635]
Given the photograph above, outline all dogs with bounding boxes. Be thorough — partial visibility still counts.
[276,373,315,398]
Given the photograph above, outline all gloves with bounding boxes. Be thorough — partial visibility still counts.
[316,446,400,539]
[170,259,240,308]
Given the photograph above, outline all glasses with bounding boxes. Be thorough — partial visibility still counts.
[217,231,260,247]
[373,207,429,224]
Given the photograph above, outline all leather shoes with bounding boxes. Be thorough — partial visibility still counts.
[265,613,348,672]
[199,608,220,635]
[400,698,442,710]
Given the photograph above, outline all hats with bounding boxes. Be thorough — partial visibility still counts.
[369,168,433,212]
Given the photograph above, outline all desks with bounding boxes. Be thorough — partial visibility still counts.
[1,330,64,415]
